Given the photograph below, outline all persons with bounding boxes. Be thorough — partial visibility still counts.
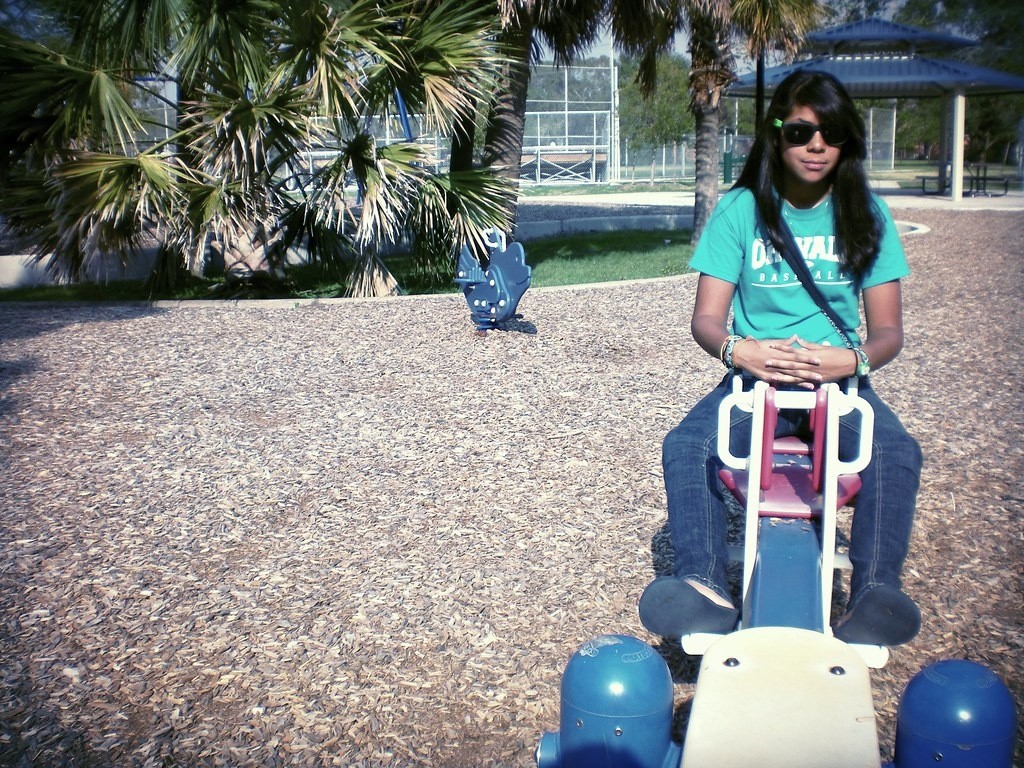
[638,66,923,644]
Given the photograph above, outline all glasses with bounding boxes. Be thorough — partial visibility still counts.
[773,117,849,147]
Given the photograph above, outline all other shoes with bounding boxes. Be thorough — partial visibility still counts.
[639,576,740,639]
[834,584,921,647]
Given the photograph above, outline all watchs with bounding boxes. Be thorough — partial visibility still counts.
[854,349,870,377]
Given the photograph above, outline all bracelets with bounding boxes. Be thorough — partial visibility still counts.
[850,348,858,378]
[720,334,742,369]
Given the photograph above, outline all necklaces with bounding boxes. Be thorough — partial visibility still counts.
[782,201,828,238]
